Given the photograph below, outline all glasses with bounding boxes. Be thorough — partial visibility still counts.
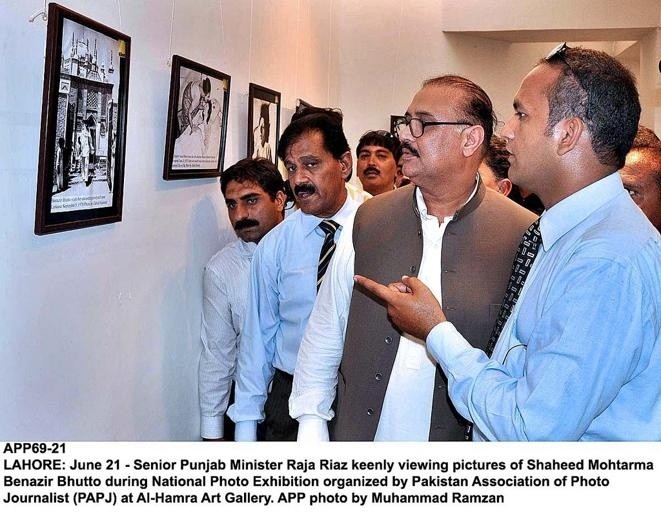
[391,115,473,139]
[372,129,396,140]
[545,41,592,120]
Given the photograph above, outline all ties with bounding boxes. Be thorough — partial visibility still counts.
[316,219,339,294]
[463,217,541,442]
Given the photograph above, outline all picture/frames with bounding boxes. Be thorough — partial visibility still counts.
[248,82,281,168]
[162,55,231,181]
[34,2,132,236]
[391,116,407,138]
[296,99,312,114]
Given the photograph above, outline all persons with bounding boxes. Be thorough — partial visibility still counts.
[225,113,366,443]
[196,152,289,444]
[284,74,544,442]
[349,41,660,443]
[252,104,273,163]
[54,135,66,190]
[181,76,212,135]
[190,98,215,152]
[278,105,346,218]
[351,124,660,233]
[78,122,97,183]
